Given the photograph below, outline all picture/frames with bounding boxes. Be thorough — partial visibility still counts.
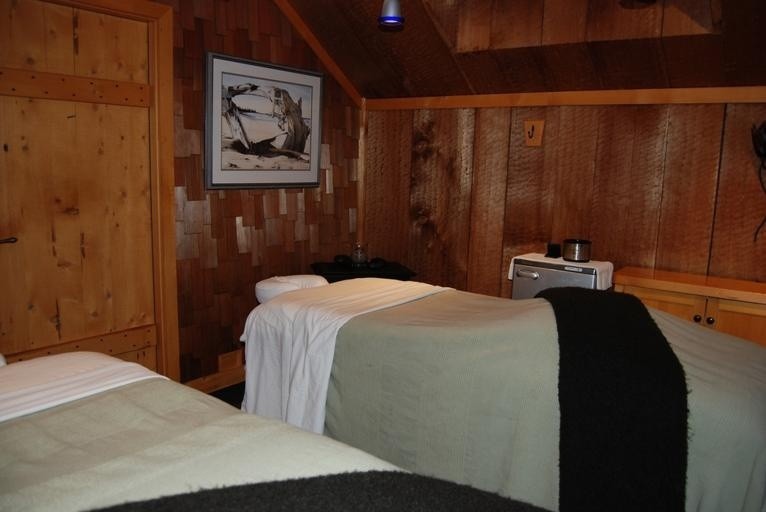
[203,50,324,191]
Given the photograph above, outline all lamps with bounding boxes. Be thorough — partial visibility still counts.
[377,0,405,28]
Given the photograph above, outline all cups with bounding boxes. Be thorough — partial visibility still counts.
[351,239,367,266]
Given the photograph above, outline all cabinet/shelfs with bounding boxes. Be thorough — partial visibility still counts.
[613,265,765,351]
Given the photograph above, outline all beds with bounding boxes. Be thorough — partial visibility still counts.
[233,275,764,512]
[0,351,559,512]
